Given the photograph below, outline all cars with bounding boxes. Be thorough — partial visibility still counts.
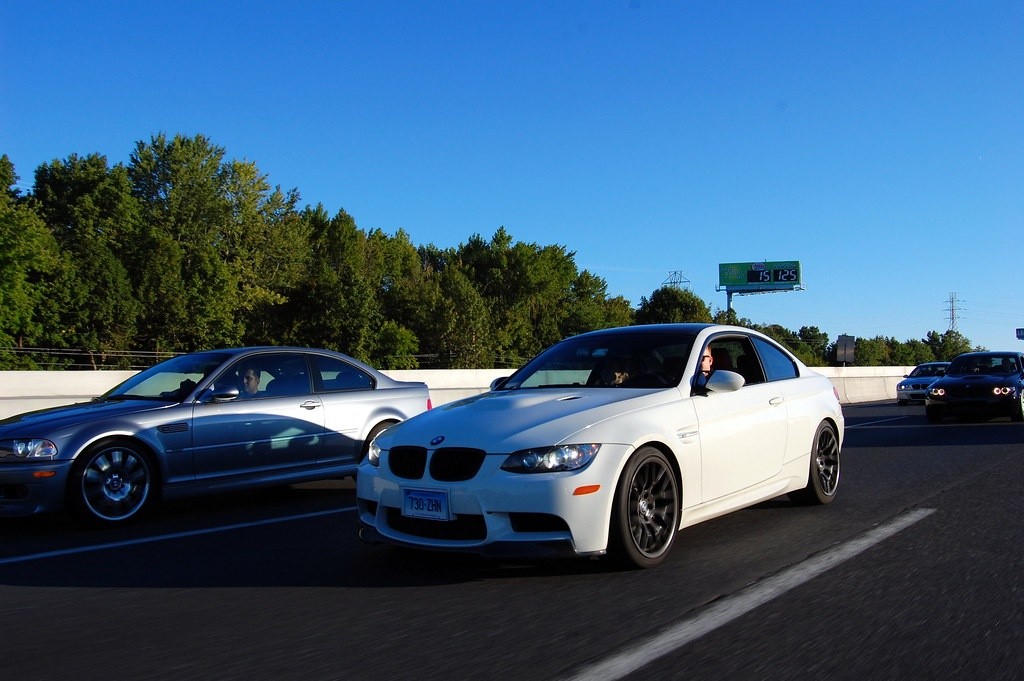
[0,343,433,532]
[353,321,845,570]
[896,362,966,406]
[923,351,1024,422]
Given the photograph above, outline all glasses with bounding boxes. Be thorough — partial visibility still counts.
[701,356,709,362]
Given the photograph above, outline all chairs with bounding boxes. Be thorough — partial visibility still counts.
[962,361,1018,375]
[592,345,765,397]
[166,370,373,407]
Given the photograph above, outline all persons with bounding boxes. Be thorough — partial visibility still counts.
[238,360,264,398]
[675,340,716,394]
[998,358,1017,373]
